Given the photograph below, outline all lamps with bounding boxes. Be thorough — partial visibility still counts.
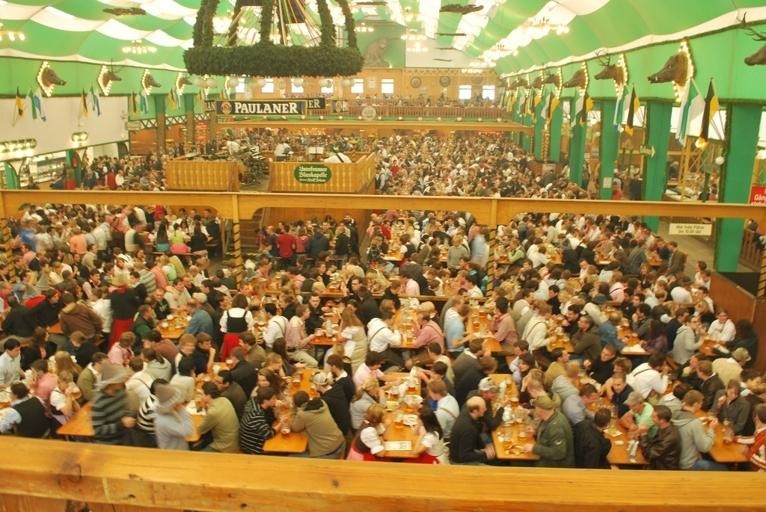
[193,75,356,89]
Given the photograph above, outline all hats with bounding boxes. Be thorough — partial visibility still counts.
[623,390,645,406]
[311,373,328,386]
[591,293,607,304]
[154,383,186,414]
[732,347,752,363]
[529,393,561,410]
[94,363,130,387]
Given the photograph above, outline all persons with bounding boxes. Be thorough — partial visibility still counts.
[0,91,765,469]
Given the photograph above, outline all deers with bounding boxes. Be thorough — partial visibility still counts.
[103,58,123,88]
[539,65,560,88]
[179,73,192,90]
[512,74,527,89]
[735,12,766,67]
[593,48,623,85]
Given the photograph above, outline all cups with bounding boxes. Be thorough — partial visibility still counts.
[383,378,423,429]
[192,357,233,413]
[721,418,735,444]
[497,378,529,454]
[577,367,645,451]
[275,367,327,440]
[162,315,174,334]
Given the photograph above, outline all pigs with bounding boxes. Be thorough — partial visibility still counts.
[144,73,162,90]
[645,54,687,85]
[526,76,542,90]
[41,67,67,88]
[562,68,585,90]
